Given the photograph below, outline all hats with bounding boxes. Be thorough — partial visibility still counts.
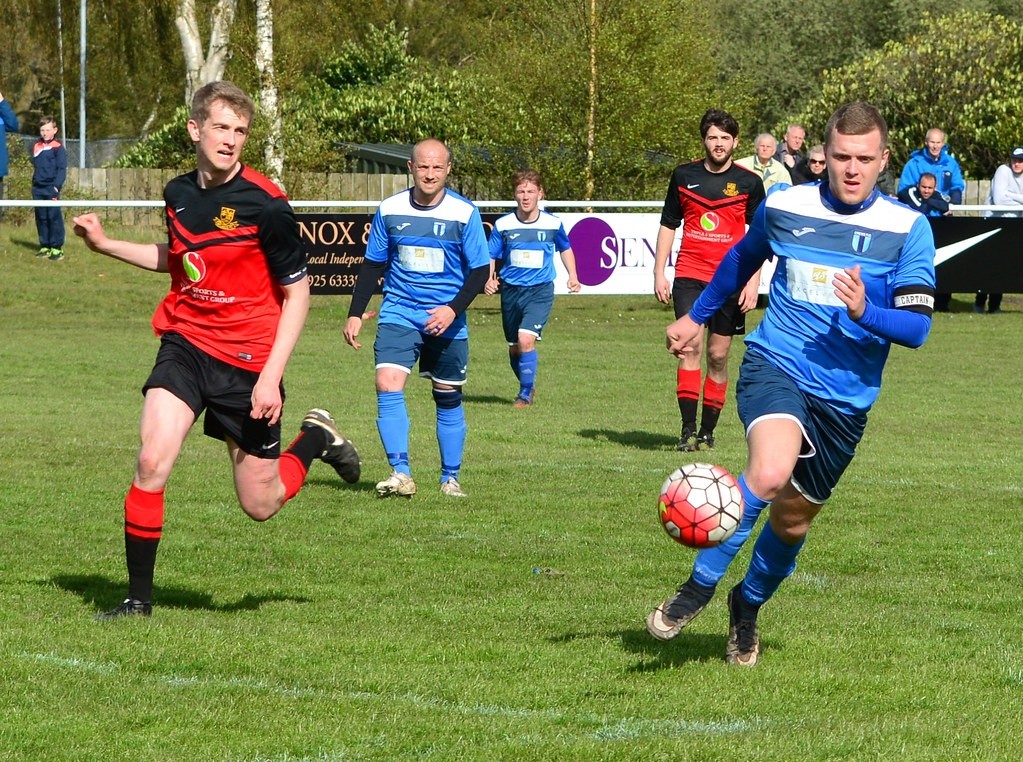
[1010,147,1023,159]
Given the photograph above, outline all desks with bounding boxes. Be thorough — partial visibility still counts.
[928,217,1023,294]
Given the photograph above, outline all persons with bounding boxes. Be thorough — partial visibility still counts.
[644,103,934,661]
[340,139,492,498]
[0,92,18,203]
[655,108,1023,458]
[32,117,68,259]
[77,81,362,620]
[484,170,581,408]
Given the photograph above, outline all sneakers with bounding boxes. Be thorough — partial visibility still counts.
[677,426,714,452]
[439,477,467,497]
[303,409,361,484]
[513,397,529,407]
[376,468,417,497]
[35,247,64,261]
[646,576,717,641]
[727,580,759,666]
[98,596,151,618]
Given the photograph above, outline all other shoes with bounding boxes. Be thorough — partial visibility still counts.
[973,303,985,314]
[988,307,1002,314]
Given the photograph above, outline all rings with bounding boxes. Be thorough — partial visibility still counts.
[436,326,441,330]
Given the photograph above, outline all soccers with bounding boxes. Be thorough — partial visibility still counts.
[657,462,745,549]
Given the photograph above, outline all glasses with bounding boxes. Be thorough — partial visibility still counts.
[807,157,826,166]
